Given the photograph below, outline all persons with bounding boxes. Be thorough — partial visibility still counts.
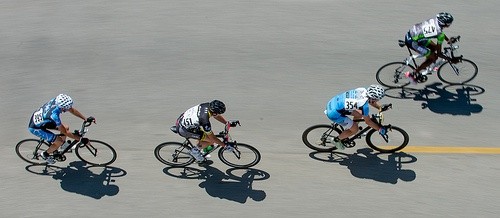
[326,84,388,150]
[405,12,459,86]
[27,94,96,164]
[176,99,237,164]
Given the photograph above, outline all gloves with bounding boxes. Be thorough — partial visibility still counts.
[378,127,387,135]
[226,120,236,127]
[79,137,90,144]
[448,37,457,44]
[87,116,96,123]
[450,57,460,64]
[224,145,233,152]
[381,104,388,111]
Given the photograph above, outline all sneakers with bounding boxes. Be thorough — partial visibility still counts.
[189,148,205,162]
[59,141,81,150]
[333,136,356,148]
[41,151,56,164]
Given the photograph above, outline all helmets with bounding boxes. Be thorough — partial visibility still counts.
[436,12,453,27]
[366,84,385,101]
[56,94,73,110]
[208,100,226,114]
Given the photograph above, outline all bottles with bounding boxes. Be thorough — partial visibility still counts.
[351,125,362,137]
[203,144,214,153]
[61,140,71,150]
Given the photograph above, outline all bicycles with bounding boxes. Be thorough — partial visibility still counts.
[153,120,261,169]
[376,35,478,89]
[302,103,409,153]
[14,117,117,167]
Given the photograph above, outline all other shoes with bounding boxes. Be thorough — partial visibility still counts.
[407,68,427,83]
